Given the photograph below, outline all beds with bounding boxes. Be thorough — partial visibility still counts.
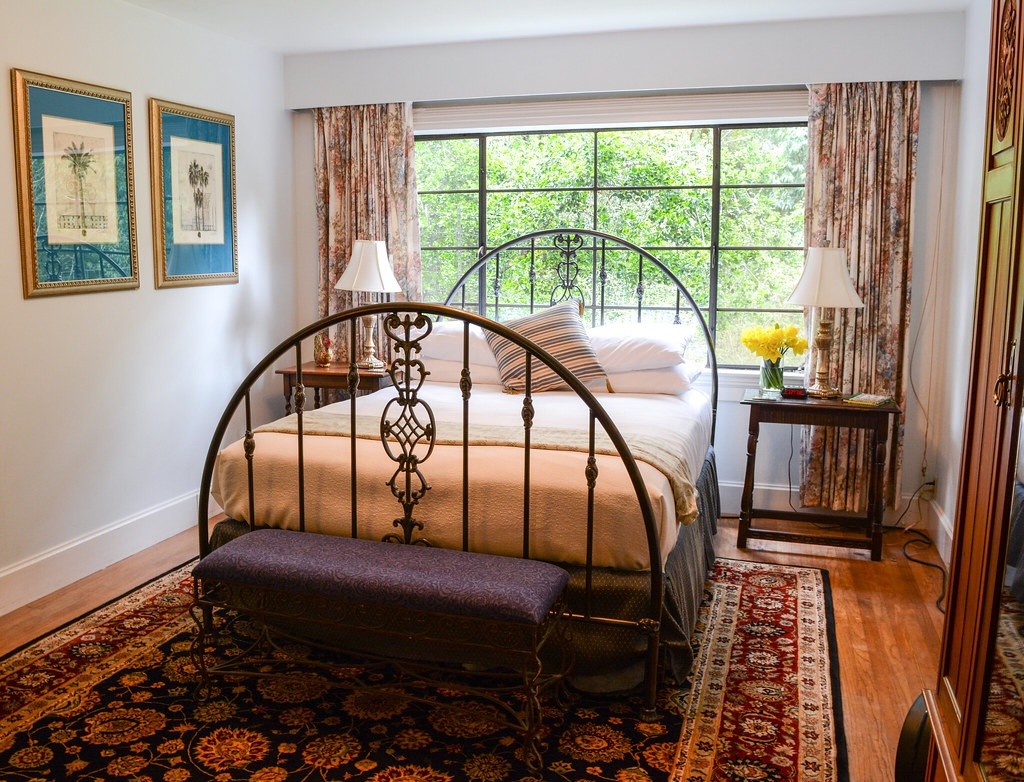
[188,377,722,723]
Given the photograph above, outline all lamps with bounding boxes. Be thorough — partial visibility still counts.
[784,247,866,400]
[333,240,403,369]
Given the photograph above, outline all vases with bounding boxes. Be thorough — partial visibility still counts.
[757,358,784,400]
[317,347,333,367]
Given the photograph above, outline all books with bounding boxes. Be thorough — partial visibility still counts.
[841,391,891,407]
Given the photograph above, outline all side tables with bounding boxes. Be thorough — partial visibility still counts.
[736,386,903,562]
[275,360,404,417]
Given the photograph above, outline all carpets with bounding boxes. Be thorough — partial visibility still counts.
[978,586,1024,782]
[1,555,851,782]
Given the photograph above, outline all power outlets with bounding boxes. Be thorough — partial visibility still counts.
[932,475,938,500]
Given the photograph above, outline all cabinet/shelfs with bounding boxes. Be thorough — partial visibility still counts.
[921,1,1024,782]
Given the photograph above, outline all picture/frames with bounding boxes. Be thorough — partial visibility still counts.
[11,67,140,300]
[148,97,239,290]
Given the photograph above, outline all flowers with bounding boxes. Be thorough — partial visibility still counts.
[322,337,331,348]
[741,322,808,389]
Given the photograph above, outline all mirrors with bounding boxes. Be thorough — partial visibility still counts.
[977,385,1024,782]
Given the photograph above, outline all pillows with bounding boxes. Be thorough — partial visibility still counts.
[396,320,498,368]
[586,322,696,374]
[396,356,503,385]
[480,297,607,395]
[561,357,702,396]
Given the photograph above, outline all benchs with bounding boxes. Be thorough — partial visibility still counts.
[191,528,581,774]
